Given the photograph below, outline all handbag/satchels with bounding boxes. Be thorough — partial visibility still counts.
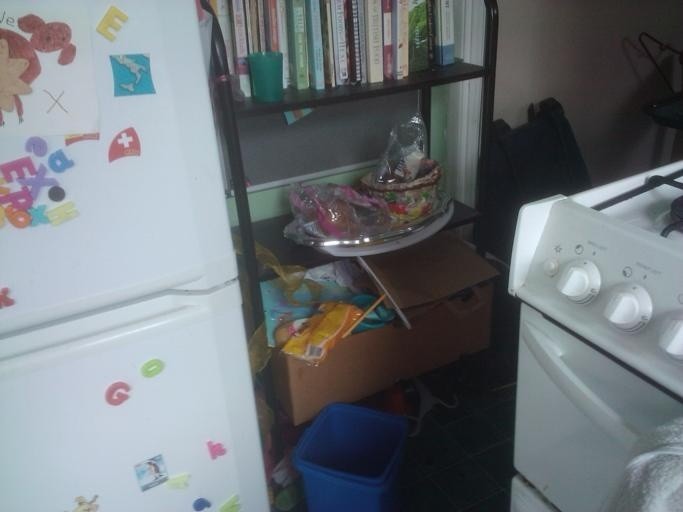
[359,160,444,224]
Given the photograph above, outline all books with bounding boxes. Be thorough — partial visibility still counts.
[204,0,460,89]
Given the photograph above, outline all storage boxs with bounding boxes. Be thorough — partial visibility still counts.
[263,233,500,424]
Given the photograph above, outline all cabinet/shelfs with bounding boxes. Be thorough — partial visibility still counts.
[203,2,501,422]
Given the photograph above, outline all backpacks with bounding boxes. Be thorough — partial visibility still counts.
[476,96,591,218]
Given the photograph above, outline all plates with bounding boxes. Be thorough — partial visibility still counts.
[269,189,456,246]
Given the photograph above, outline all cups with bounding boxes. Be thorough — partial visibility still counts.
[247,49,287,103]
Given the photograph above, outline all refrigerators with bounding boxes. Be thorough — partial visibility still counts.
[1,1,277,512]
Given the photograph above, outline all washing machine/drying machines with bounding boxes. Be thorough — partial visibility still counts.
[506,158,682,512]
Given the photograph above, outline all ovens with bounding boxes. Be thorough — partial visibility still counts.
[508,307,681,509]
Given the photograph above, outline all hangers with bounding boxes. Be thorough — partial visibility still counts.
[639,31,682,95]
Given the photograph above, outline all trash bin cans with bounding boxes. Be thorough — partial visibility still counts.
[293,402,410,512]
